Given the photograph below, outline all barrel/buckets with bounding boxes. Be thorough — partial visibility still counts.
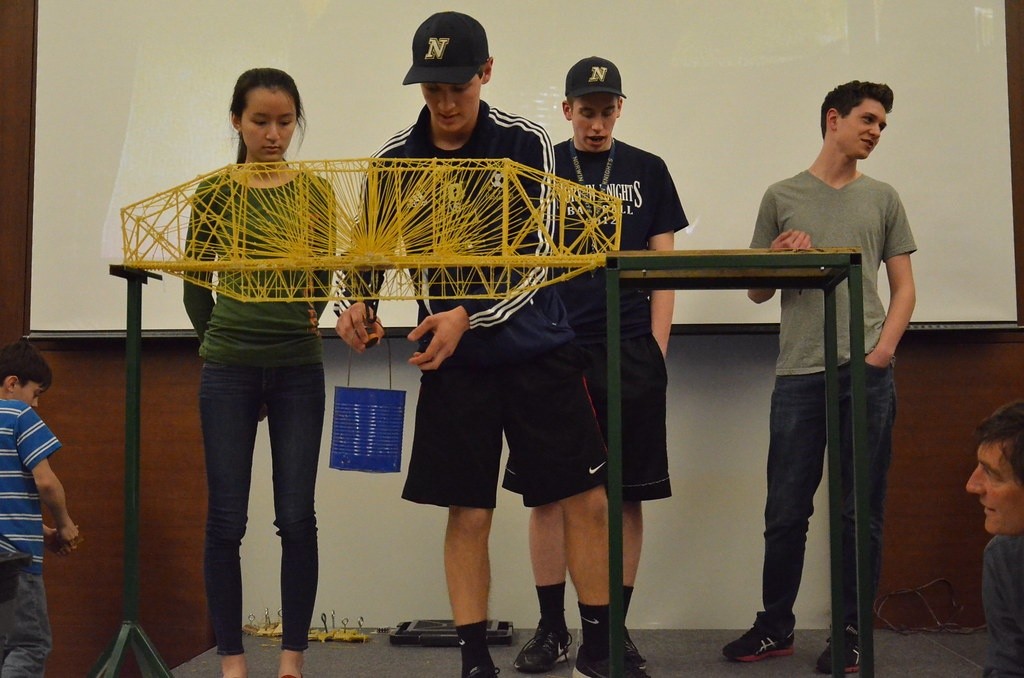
[329,386,407,475]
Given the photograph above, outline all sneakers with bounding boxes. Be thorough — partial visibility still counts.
[622,625,647,669]
[572,646,652,678]
[464,664,501,678]
[816,623,860,674]
[513,619,572,673]
[722,621,795,661]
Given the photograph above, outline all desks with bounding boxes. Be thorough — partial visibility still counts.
[605,249,876,678]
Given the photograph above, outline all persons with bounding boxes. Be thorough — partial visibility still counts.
[502,56,690,673]
[722,80,917,674]
[965,399,1024,678]
[0,340,85,678]
[183,68,337,678]
[333,11,651,678]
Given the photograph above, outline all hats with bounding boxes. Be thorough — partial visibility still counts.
[566,57,627,99]
[403,12,489,84]
